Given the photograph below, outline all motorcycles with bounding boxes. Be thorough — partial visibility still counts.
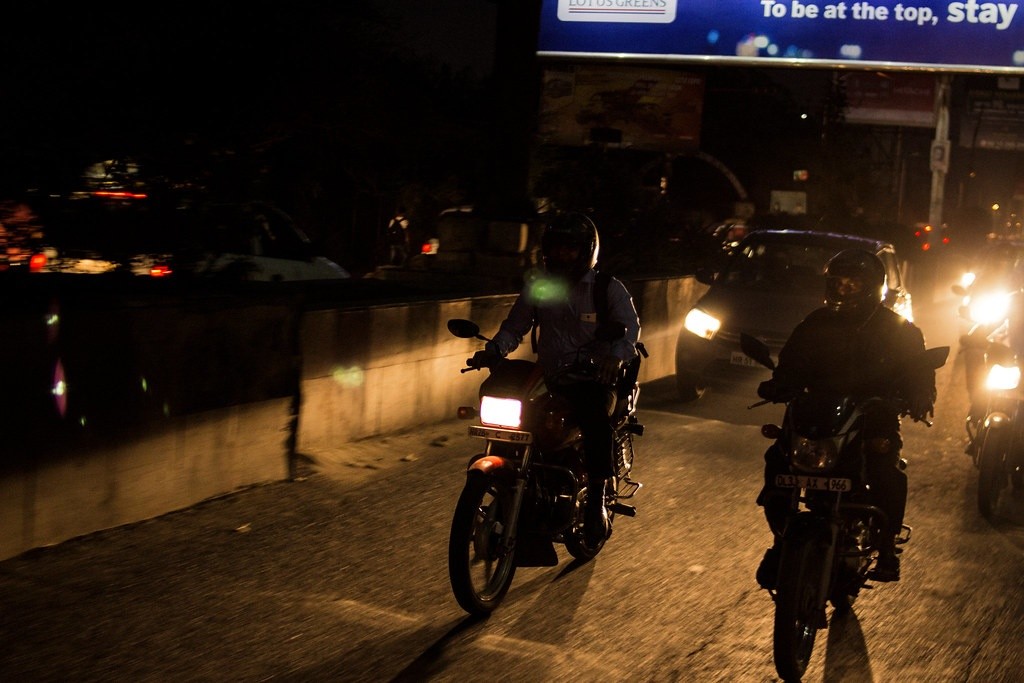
[442,318,644,612]
[736,334,950,683]
[958,335,1023,515]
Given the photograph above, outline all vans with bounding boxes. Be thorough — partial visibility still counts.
[440,144,755,286]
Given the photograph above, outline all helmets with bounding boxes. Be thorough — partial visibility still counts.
[540,211,599,280]
[824,249,885,319]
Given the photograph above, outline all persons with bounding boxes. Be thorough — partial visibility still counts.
[758,247,939,582]
[469,208,643,550]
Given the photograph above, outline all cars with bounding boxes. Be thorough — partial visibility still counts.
[673,230,914,403]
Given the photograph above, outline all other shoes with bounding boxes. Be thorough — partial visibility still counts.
[755,549,780,590]
[583,477,609,548]
[872,553,901,581]
